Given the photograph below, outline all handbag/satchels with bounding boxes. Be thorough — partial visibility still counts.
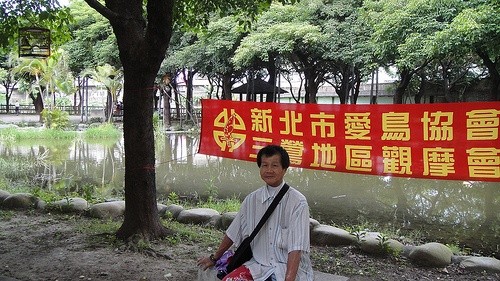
[217,237,253,280]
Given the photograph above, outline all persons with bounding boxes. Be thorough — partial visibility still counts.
[114,102,123,115]
[196,145,314,281]
[15,101,20,115]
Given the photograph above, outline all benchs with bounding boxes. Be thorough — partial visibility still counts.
[198,252,349,281]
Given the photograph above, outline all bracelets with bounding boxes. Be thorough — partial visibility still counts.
[209,253,216,264]
